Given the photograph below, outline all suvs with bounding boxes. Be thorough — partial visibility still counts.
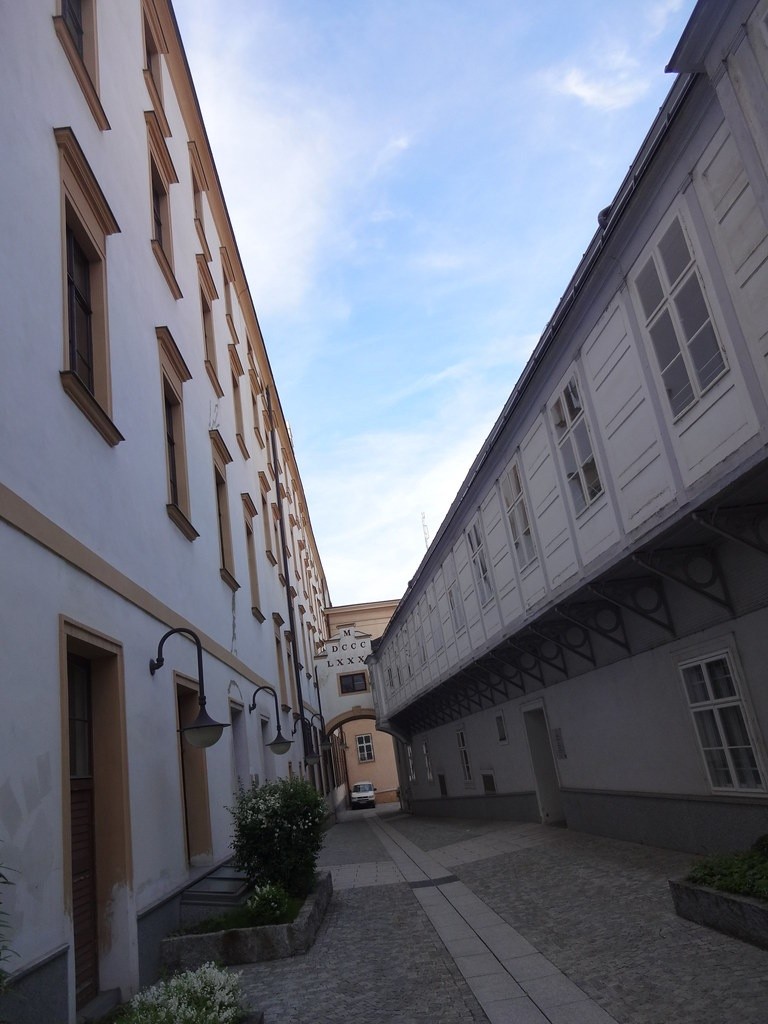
[350,782,377,810]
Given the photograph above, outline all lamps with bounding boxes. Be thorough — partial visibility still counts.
[338,740,346,750]
[337,731,350,751]
[291,717,323,766]
[149,627,232,748]
[310,714,332,751]
[248,685,295,755]
